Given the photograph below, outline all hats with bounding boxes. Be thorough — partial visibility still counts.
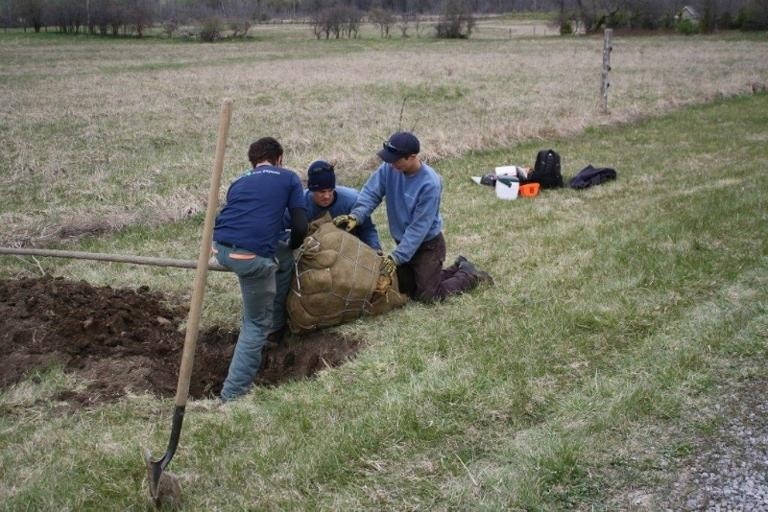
[308,160,335,190]
[377,132,420,163]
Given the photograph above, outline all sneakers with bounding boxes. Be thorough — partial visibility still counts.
[453,254,495,287]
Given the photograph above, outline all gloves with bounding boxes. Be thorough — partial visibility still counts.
[380,254,397,275]
[332,215,357,232]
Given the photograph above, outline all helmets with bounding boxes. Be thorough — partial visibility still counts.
[520,182,541,197]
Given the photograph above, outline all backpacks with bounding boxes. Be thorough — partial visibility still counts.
[569,163,617,189]
[531,149,564,188]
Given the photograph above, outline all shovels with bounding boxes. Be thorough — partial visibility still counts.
[144,98,236,511]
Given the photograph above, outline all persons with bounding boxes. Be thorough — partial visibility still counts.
[284,159,385,261]
[210,136,310,409]
[331,131,496,308]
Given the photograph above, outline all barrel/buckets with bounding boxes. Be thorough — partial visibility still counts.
[495,166,516,175]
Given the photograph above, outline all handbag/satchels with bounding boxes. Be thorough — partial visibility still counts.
[480,164,533,185]
